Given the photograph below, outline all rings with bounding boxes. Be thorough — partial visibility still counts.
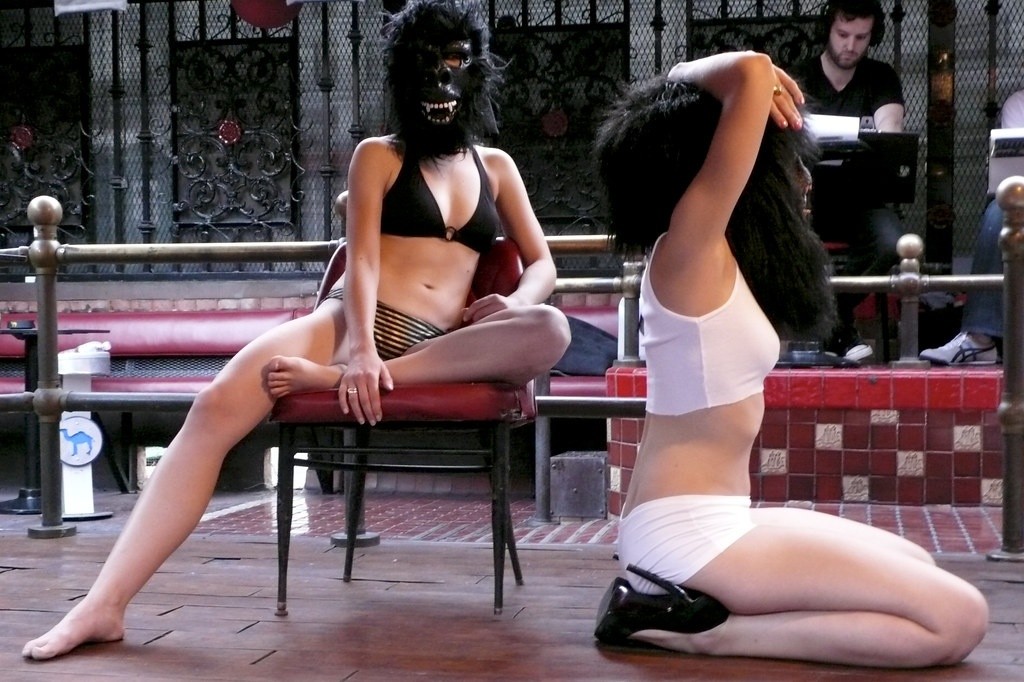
[773,83,782,94]
[346,388,357,394]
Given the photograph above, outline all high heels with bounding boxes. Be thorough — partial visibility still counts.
[593,563,730,643]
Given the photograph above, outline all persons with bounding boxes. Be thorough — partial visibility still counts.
[20,0,572,661]
[784,0,1024,366]
[592,49,992,670]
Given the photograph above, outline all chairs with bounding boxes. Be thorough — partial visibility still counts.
[271,239,537,617]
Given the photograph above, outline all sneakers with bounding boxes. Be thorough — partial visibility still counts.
[827,325,874,361]
[920,333,999,366]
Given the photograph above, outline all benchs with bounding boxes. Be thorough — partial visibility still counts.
[0,306,620,495]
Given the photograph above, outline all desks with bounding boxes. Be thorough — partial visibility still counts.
[0,330,110,514]
